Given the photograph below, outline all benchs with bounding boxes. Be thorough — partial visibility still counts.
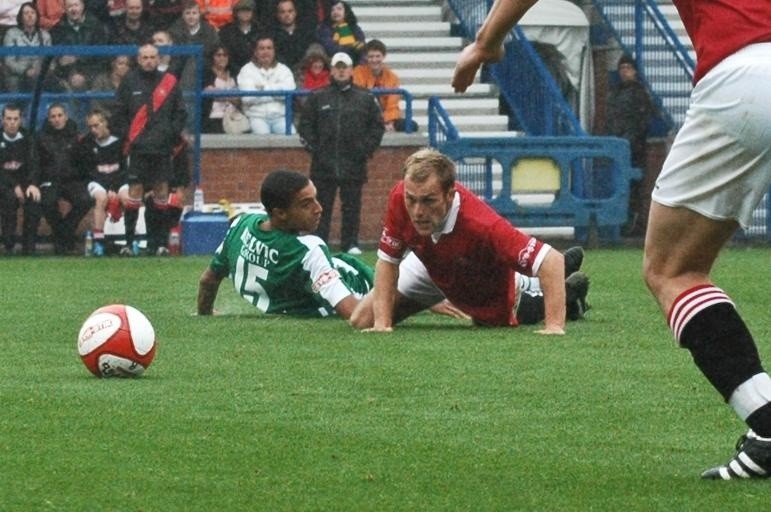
[179,201,268,257]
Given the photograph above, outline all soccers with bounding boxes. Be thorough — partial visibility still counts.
[77,304,157,379]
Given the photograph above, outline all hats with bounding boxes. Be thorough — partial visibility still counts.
[330,52,352,66]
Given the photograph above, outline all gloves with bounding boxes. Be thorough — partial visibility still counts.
[106,198,120,222]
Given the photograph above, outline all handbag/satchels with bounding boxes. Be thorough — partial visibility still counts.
[222,101,249,135]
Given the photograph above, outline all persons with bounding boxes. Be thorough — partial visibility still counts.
[348,148,587,336]
[78,109,129,256]
[113,44,190,257]
[1,0,401,133]
[598,51,652,234]
[450,0,771,482]
[33,102,96,259]
[193,167,468,320]
[140,129,199,257]
[296,52,387,253]
[0,104,40,255]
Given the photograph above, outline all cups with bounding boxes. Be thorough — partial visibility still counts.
[169,228,181,256]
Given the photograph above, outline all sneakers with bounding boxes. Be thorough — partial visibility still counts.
[700,429,770,480]
[119,245,169,257]
[347,247,361,255]
[563,246,589,320]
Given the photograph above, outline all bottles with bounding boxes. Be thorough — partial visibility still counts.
[84,231,94,256]
[194,186,205,213]
